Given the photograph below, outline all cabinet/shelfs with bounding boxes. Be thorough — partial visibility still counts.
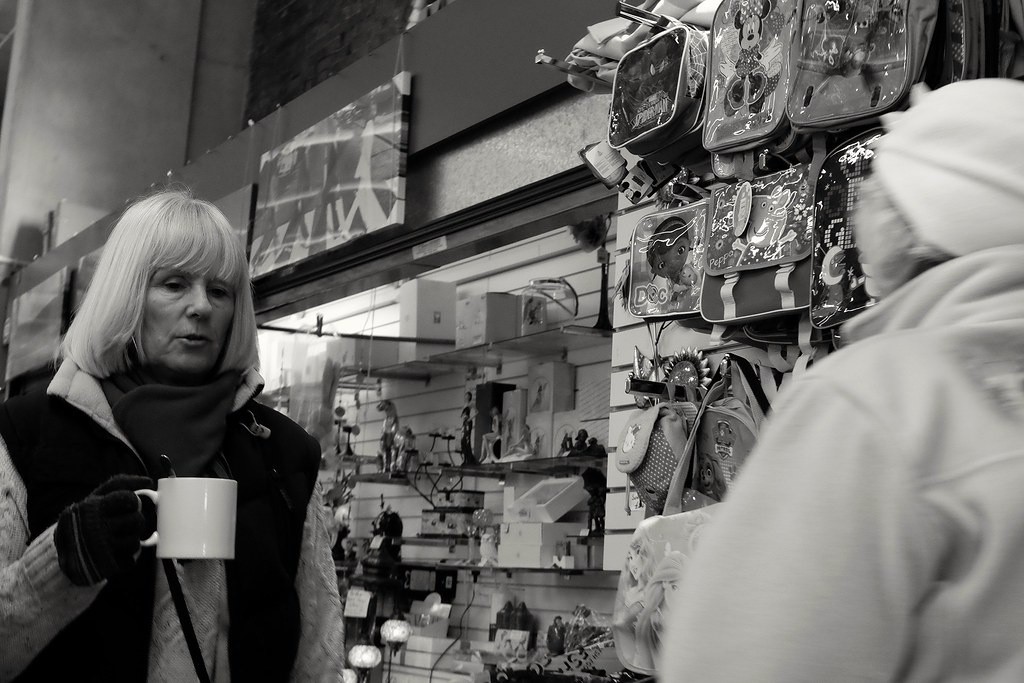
[253,324,612,576]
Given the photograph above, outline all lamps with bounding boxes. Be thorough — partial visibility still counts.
[342,608,412,683]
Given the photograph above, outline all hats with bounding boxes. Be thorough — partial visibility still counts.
[868,76,1024,253]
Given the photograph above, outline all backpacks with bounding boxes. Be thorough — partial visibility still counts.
[605,0,1024,514]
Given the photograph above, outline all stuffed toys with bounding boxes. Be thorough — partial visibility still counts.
[665,261,701,297]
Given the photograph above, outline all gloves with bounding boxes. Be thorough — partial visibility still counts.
[53,474,157,589]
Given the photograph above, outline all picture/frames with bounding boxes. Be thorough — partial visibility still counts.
[4,72,412,381]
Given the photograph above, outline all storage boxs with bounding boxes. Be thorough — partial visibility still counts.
[327,280,588,569]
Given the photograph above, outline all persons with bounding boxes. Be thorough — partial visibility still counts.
[646,216,693,290]
[500,419,533,457]
[478,408,500,461]
[612,531,690,669]
[587,485,605,531]
[614,42,666,111]
[459,391,479,468]
[652,78,1024,683]
[0,191,344,683]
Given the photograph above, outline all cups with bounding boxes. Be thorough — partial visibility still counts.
[134,477,237,560]
